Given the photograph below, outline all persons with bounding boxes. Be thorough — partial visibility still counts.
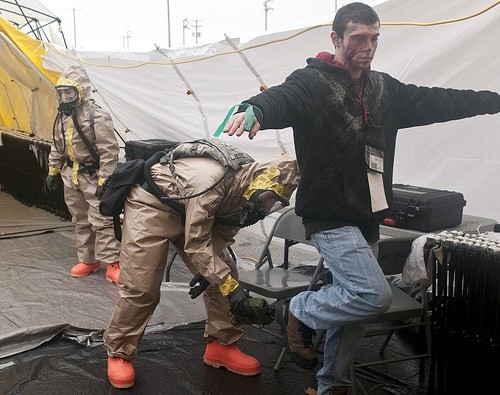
[222,2,500,395]
[103,137,300,388]
[46,65,120,287]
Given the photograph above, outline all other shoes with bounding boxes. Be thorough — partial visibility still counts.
[107,354,136,389]
[203,338,261,376]
[70,259,102,278]
[105,261,125,288]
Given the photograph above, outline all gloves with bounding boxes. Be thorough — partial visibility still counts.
[226,287,254,317]
[45,175,58,192]
[188,271,210,300]
[94,186,103,199]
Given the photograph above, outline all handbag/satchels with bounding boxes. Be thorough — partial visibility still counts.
[98,158,145,243]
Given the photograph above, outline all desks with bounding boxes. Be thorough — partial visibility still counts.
[378,216,498,275]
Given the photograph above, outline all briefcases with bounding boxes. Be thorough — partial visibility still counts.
[379,183,467,232]
[124,138,181,162]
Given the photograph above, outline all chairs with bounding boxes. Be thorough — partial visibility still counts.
[242,206,441,395]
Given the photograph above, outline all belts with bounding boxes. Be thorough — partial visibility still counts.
[67,156,99,172]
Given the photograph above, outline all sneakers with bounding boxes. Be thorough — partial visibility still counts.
[274,298,319,370]
